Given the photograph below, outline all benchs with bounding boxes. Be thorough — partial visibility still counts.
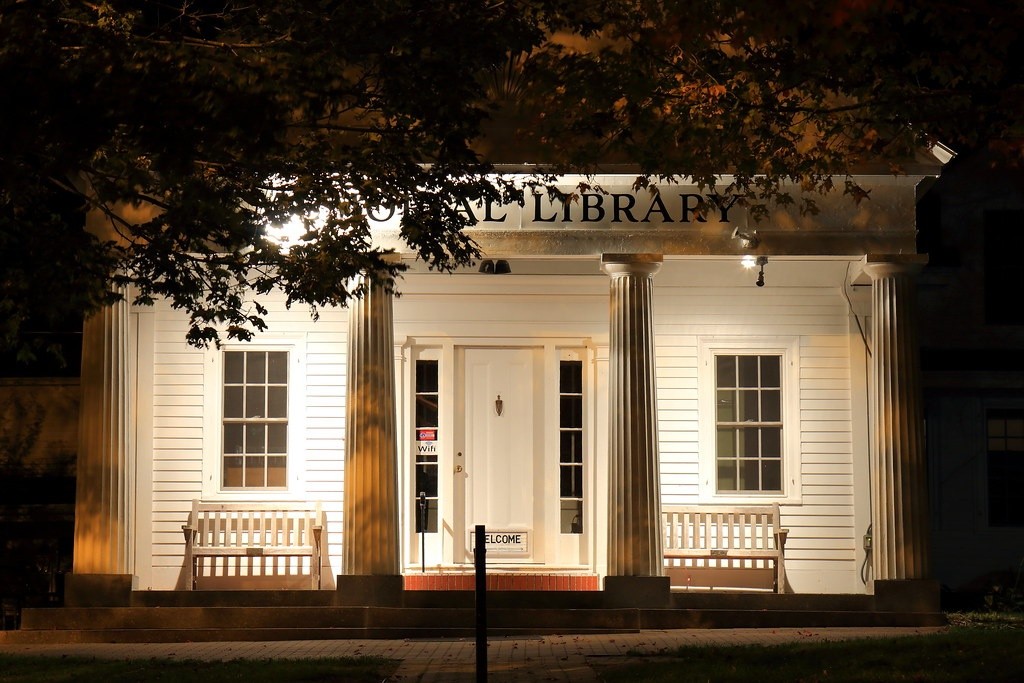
[660,502,789,594]
[180,499,323,590]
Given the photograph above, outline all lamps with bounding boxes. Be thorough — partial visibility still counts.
[476,258,511,274]
[730,226,760,249]
[755,257,768,287]
